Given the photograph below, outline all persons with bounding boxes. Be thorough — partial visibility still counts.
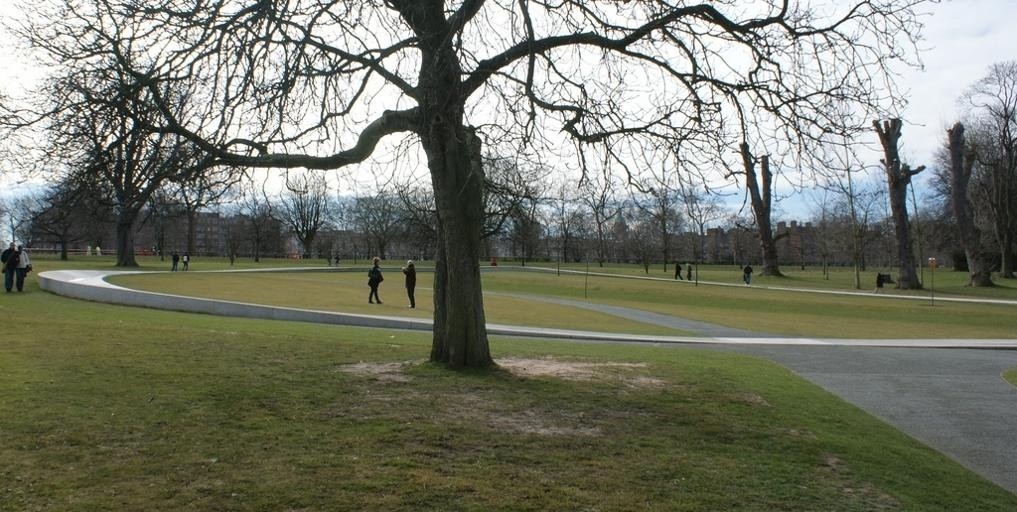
[172,251,180,271]
[15,246,30,292]
[402,260,416,308]
[181,252,189,272]
[334,253,340,264]
[1,242,20,292]
[875,272,884,294]
[687,265,692,280]
[368,256,384,303]
[743,262,753,286]
[675,263,683,280]
